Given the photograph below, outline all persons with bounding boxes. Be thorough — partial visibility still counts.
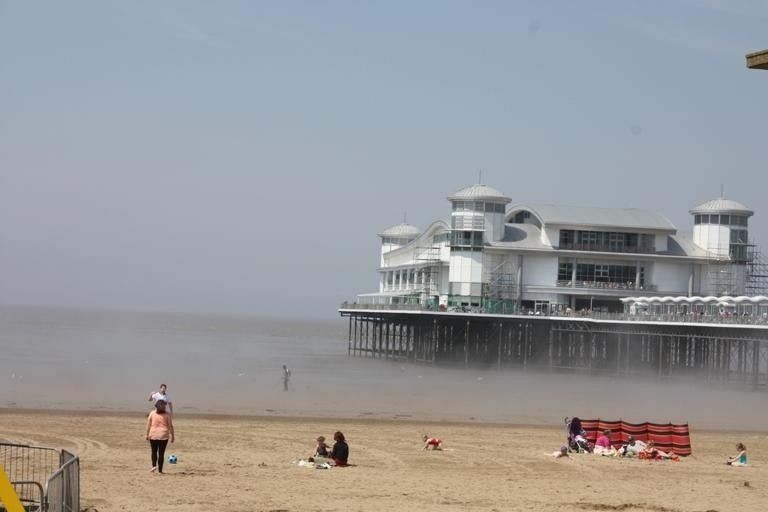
[566,417,589,450]
[592,428,616,456]
[281,364,292,392]
[146,399,175,475]
[724,439,750,467]
[642,438,657,458]
[626,434,679,459]
[314,435,332,458]
[422,434,444,452]
[544,446,574,459]
[448,302,767,323]
[149,381,175,420]
[328,430,349,466]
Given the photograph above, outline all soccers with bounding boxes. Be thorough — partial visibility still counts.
[168,455,177,463]
